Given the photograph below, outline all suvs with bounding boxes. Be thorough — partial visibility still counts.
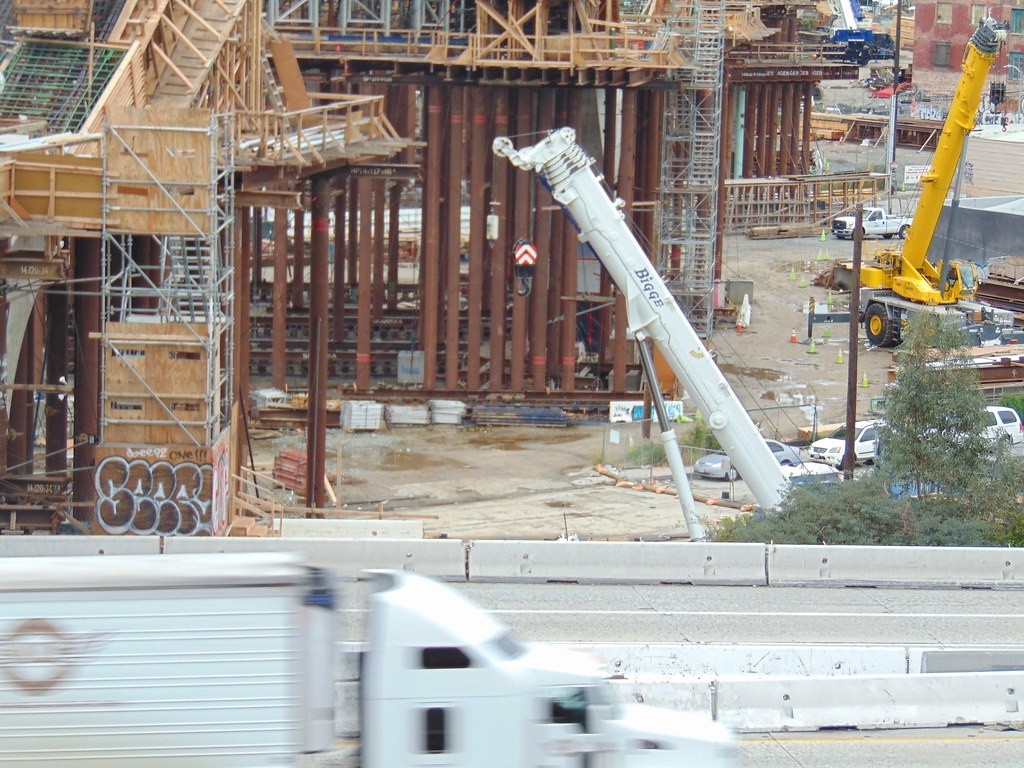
[808,419,898,471]
[953,406,1023,455]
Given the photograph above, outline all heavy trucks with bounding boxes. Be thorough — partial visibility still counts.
[0,549,739,768]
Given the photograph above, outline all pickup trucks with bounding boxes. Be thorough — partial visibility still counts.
[831,209,913,239]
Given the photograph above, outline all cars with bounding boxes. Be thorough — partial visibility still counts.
[694,438,802,481]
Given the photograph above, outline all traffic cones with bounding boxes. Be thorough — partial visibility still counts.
[901,182,906,192]
[677,414,694,423]
[858,370,870,388]
[806,336,821,354]
[819,228,828,242]
[788,327,798,343]
[823,157,830,171]
[798,274,808,288]
[695,408,703,421]
[834,346,846,364]
[735,313,744,332]
[788,268,797,281]
[826,288,834,305]
[820,323,832,338]
[823,248,831,260]
[816,248,823,261]
[838,136,844,145]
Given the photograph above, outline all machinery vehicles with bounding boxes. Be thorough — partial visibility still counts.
[806,16,1017,348]
[803,1,897,62]
